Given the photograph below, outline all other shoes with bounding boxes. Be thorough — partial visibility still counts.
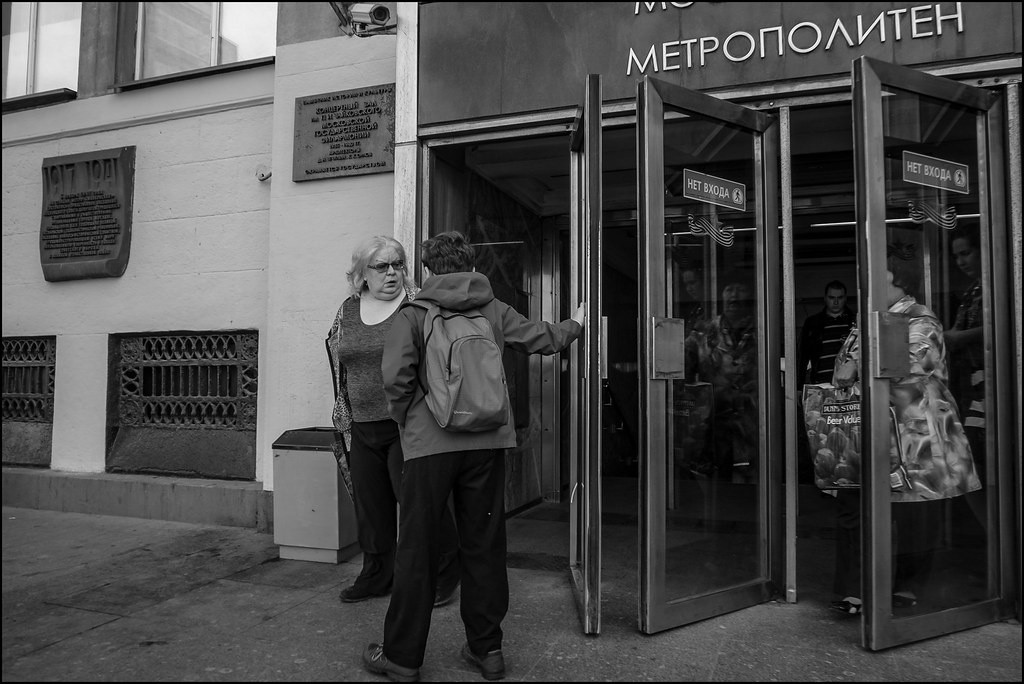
[433,579,452,606]
[338,579,387,603]
[891,593,919,608]
[828,600,862,615]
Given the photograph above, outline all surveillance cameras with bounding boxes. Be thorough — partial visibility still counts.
[348,4,390,28]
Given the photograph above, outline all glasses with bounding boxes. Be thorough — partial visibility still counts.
[367,259,404,273]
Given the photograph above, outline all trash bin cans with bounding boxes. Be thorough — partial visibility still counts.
[272,426,362,564]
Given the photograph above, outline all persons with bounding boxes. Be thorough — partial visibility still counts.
[797,281,855,407]
[828,244,983,615]
[685,274,757,569]
[682,266,723,338]
[943,227,994,536]
[361,233,586,683]
[324,237,461,605]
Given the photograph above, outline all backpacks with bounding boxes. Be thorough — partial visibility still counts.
[397,298,511,434]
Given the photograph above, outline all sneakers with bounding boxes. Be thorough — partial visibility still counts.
[461,641,506,680]
[362,641,420,682]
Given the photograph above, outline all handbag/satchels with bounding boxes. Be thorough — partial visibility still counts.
[801,376,915,491]
